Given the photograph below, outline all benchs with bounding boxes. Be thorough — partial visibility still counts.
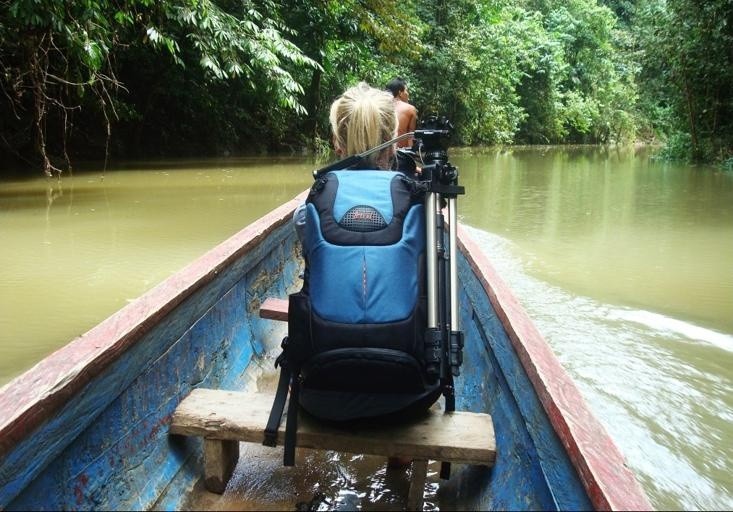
[169,387,498,512]
[258,297,293,322]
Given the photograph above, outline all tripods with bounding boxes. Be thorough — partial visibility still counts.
[312,128,466,377]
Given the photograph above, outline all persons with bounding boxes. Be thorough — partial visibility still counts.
[386,80,422,173]
[287,82,446,465]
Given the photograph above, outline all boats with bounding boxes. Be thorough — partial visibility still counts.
[0,186,654,511]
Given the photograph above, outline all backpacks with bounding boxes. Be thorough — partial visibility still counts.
[300,165,429,397]
